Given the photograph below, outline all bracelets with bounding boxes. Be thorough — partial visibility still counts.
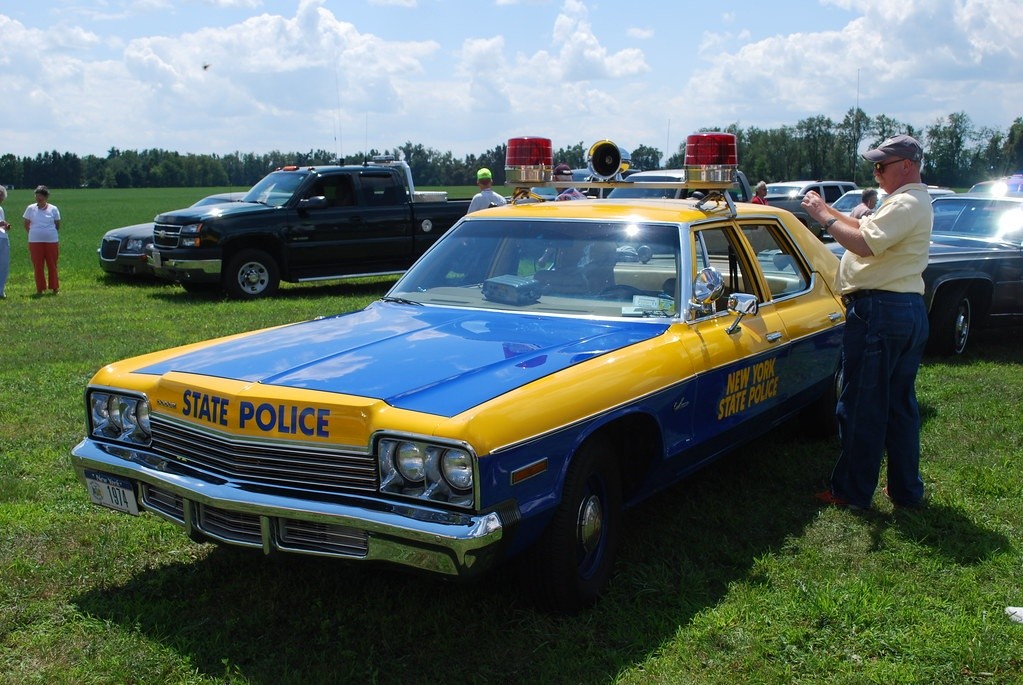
[825,219,837,234]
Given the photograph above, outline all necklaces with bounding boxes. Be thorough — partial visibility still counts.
[42,202,47,209]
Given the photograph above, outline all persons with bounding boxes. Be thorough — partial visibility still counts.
[751,181,768,206]
[538,166,592,267]
[850,188,879,219]
[467,168,507,215]
[23,186,60,295]
[0,185,12,299]
[800,134,934,513]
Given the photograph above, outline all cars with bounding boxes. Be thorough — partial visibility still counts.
[69,130,850,616]
[508,166,1023,365]
[97,192,308,285]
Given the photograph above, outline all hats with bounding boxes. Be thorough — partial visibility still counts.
[477,168,492,179]
[552,164,573,175]
[861,134,923,162]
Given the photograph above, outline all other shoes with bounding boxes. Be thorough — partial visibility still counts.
[53,289,59,295]
[1,294,7,299]
[38,290,45,295]
[883,486,894,500]
[815,492,872,514]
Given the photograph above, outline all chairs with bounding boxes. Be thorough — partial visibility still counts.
[531,240,619,296]
[305,183,326,199]
[331,175,357,208]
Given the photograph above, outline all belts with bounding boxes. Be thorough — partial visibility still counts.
[841,289,889,308]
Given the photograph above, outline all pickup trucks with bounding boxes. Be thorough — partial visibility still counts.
[144,156,513,303]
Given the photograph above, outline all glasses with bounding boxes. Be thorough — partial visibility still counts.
[874,158,913,174]
[763,188,767,191]
